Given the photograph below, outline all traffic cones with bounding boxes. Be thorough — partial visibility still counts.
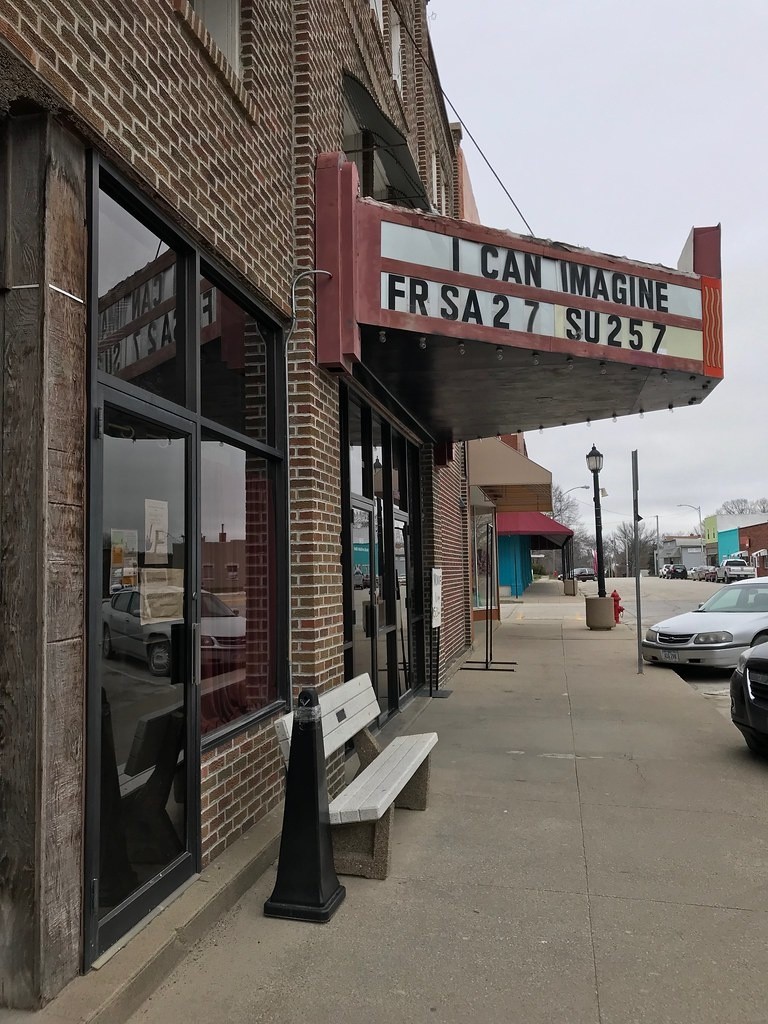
[261,686,350,925]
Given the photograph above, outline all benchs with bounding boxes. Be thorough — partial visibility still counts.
[272,673,437,881]
[118,675,249,865]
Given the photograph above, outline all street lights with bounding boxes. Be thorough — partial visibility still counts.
[677,504,704,565]
[558,485,591,526]
[373,456,385,597]
[583,442,608,597]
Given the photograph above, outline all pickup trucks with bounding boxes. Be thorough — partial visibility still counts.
[714,559,756,584]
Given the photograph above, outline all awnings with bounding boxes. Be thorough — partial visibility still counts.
[465,436,553,512]
[496,512,574,582]
[659,547,681,558]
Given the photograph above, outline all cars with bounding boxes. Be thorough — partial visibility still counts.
[352,564,407,590]
[725,638,768,757]
[557,567,596,582]
[643,575,768,673]
[100,583,247,677]
[658,563,719,582]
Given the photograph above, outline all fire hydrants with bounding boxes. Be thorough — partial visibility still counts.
[611,589,626,624]
